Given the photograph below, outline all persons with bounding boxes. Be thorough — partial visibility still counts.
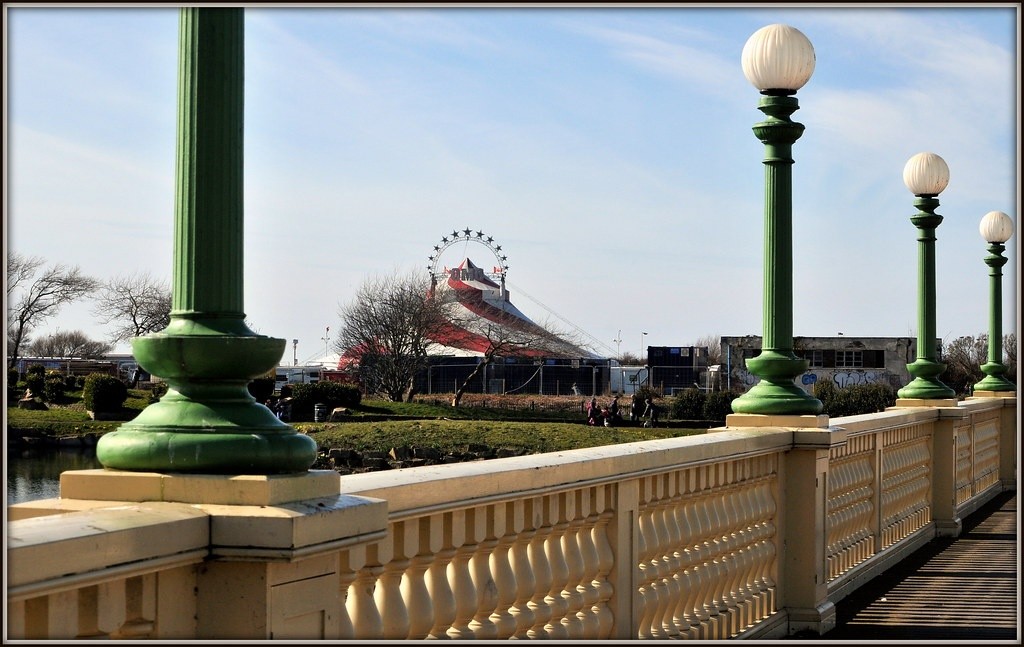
[265,398,290,422]
[642,399,660,428]
[587,395,622,427]
[630,394,640,428]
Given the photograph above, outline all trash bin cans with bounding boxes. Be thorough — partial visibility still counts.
[315,403,327,422]
